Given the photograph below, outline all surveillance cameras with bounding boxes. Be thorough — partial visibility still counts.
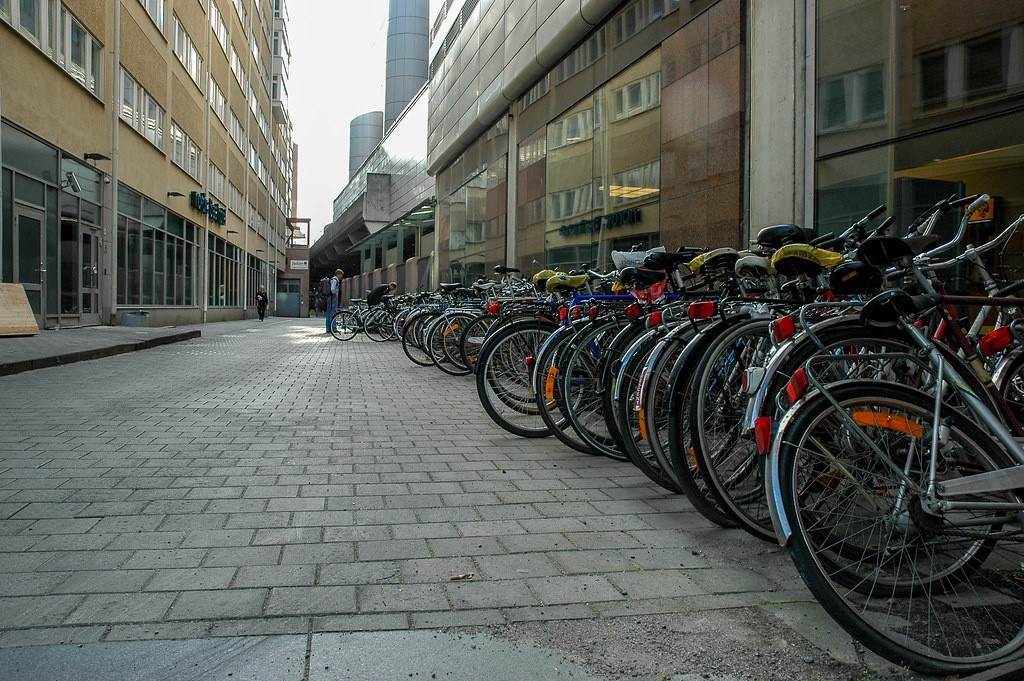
[66,172,81,193]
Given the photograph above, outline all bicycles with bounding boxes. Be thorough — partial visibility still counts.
[330,191,1024,681]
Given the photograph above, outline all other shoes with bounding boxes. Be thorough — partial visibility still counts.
[259,316,263,321]
[326,331,340,334]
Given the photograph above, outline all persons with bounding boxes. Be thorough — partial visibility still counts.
[255,284,268,321]
[367,282,397,307]
[325,268,345,334]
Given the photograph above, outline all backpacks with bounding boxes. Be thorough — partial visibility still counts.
[320,277,338,297]
